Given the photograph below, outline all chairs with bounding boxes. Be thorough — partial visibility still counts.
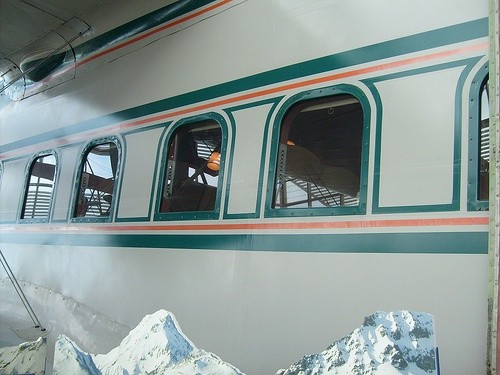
[285,145,319,208]
[319,166,356,207]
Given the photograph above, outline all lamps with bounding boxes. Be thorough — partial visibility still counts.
[207,151,221,170]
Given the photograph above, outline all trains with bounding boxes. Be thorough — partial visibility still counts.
[1,0,499,375]
